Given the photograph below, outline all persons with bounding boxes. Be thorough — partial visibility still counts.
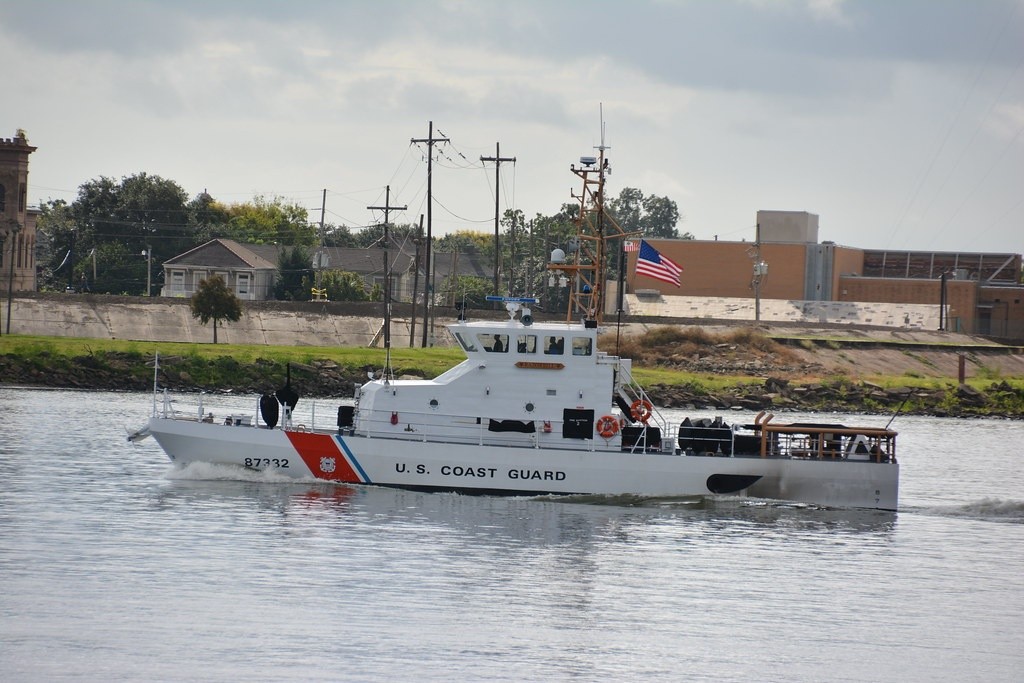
[80,273,88,292]
[518,343,526,353]
[549,337,562,354]
[206,412,214,423]
[870,445,886,463]
[824,441,837,457]
[855,441,868,453]
[493,334,503,352]
[455,296,468,320]
[226,415,232,425]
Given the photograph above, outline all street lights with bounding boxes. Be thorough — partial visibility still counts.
[316,228,335,289]
[141,248,151,297]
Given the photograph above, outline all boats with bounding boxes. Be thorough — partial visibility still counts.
[125,100,901,515]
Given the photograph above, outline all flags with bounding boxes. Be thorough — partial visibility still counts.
[635,239,683,288]
[623,241,640,252]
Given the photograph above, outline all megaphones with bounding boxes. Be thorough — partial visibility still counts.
[520,308,534,326]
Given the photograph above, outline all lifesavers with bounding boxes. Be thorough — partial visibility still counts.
[631,400,651,422]
[596,415,619,438]
